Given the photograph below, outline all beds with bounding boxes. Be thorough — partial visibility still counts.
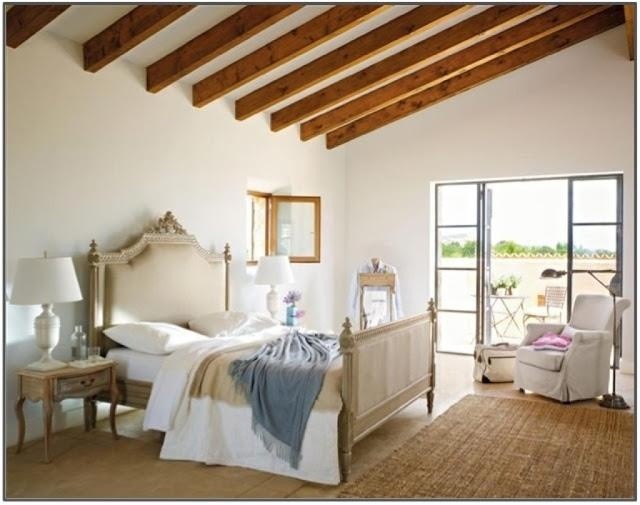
[86,209,439,487]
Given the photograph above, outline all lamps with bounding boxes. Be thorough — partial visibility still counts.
[9,251,85,373]
[539,266,631,410]
[254,251,295,323]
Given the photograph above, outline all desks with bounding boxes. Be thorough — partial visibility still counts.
[470,293,530,345]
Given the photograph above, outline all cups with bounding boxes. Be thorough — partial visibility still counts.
[91,347,100,364]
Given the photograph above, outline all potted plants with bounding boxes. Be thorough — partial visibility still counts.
[490,271,523,296]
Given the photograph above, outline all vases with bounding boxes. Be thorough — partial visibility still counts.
[286,307,297,326]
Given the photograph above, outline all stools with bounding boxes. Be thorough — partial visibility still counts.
[473,344,520,383]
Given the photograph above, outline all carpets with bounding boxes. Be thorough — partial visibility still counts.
[337,393,634,498]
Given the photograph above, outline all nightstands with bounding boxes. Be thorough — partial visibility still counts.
[14,357,121,464]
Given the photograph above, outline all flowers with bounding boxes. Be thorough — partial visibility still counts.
[283,288,307,319]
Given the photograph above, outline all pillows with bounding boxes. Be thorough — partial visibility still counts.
[532,325,577,351]
[101,309,281,358]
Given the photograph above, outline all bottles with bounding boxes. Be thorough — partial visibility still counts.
[70,325,87,361]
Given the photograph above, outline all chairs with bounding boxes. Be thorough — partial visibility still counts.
[523,285,567,337]
[513,293,632,405]
